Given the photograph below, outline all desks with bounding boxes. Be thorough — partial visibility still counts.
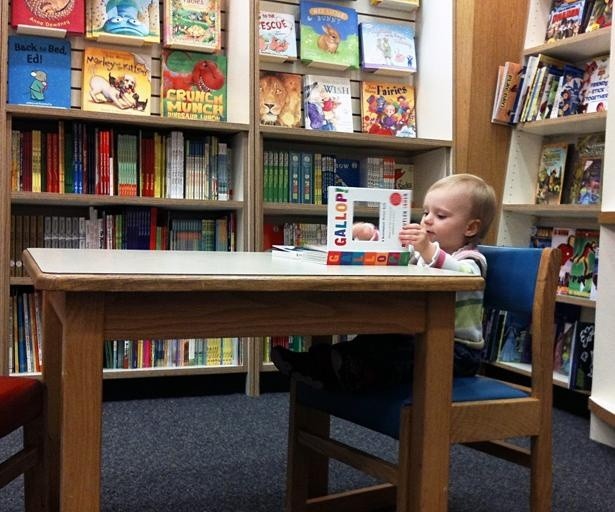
[22,245,485,512]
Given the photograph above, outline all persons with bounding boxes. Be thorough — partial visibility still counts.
[270,173,498,419]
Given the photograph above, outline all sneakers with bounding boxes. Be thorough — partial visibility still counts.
[271,343,342,392]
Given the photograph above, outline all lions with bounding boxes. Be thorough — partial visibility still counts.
[259,70,290,126]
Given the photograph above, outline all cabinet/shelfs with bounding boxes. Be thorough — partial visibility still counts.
[480,0,610,398]
[255,0,452,400]
[0,0,254,398]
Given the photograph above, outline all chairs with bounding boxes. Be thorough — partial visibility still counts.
[284,243,565,511]
